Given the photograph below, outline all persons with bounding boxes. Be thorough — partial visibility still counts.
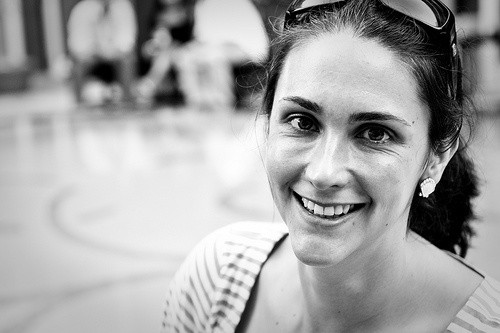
[160,0,500,333]
[64,1,271,118]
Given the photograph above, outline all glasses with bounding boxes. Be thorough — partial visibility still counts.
[283,0,458,100]
